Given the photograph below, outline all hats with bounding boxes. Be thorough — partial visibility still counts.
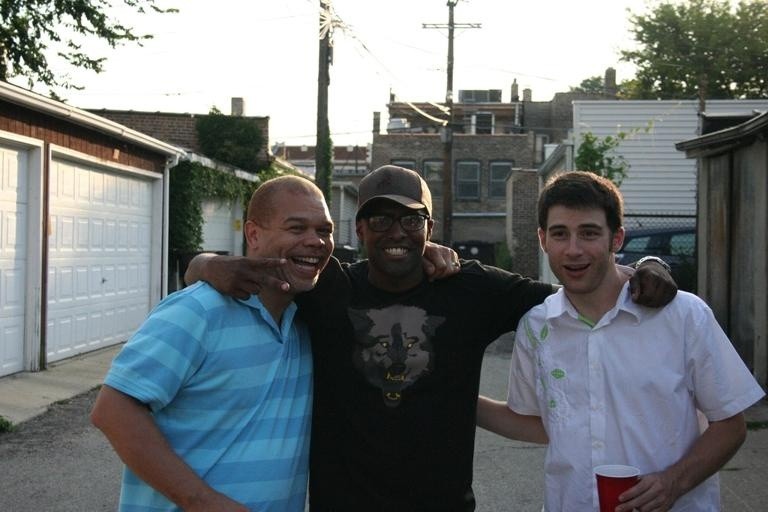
[355,165,433,225]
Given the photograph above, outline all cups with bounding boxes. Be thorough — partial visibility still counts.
[593,464,640,512]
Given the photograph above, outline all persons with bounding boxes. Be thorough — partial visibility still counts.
[472,170,767,512]
[88,176,461,512]
[183,166,678,512]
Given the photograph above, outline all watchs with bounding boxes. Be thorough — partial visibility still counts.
[634,253,673,272]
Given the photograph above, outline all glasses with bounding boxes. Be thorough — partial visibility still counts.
[357,211,429,233]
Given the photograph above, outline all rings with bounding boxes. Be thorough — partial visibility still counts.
[452,261,460,267]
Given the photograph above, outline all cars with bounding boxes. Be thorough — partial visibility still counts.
[614,224,697,296]
